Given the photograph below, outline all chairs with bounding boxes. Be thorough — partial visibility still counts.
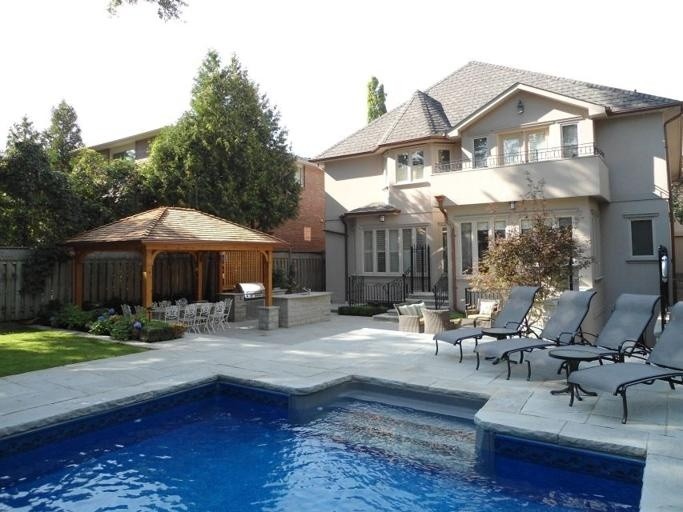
[433,286,682,424]
[393,300,461,334]
[119,298,232,335]
